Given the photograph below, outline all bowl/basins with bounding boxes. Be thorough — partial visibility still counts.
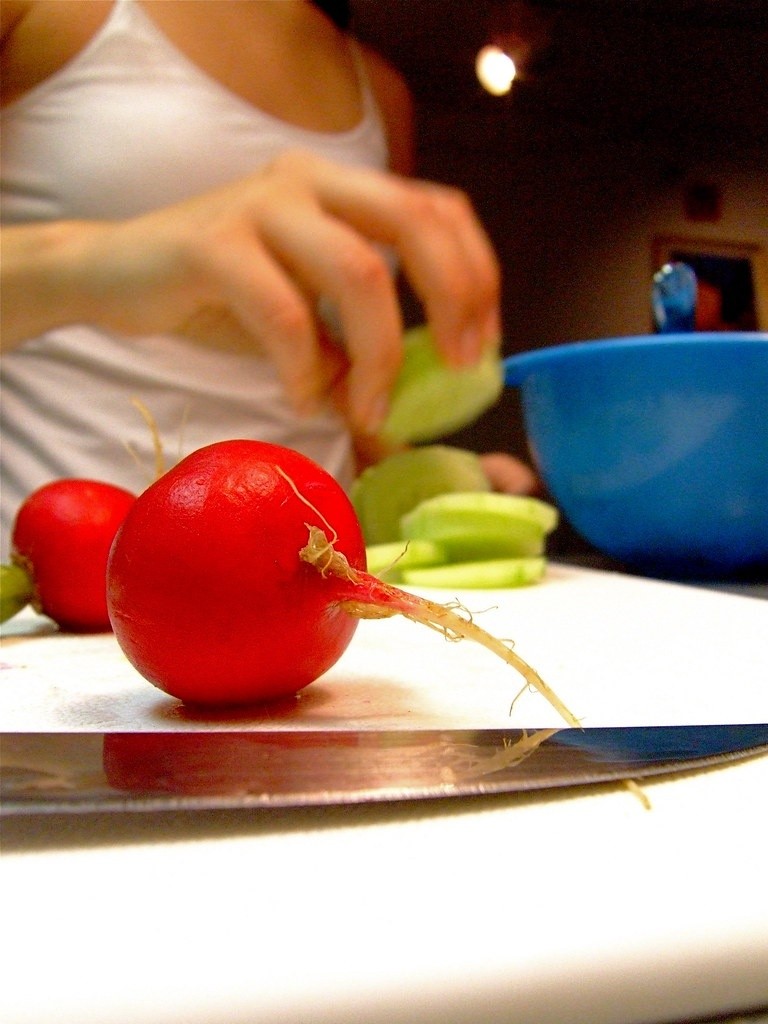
[501,330,768,575]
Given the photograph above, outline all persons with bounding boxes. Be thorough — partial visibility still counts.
[0,1,540,574]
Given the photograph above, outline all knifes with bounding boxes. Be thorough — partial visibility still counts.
[0,725,768,817]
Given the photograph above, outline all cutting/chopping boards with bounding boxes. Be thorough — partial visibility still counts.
[0,568,768,1023]
